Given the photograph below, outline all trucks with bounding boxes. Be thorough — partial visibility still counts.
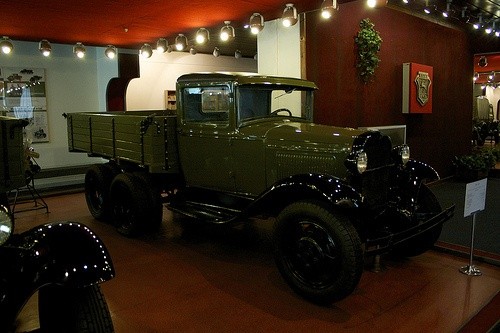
[61,70,458,307]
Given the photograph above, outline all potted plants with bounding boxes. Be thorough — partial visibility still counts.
[452,148,497,179]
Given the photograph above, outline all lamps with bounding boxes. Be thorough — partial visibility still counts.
[282,3,298,28]
[175,33,188,52]
[248,13,265,35]
[196,27,210,46]
[319,0,340,19]
[73,42,87,59]
[38,39,52,57]
[139,43,153,60]
[461,7,500,38]
[157,37,169,55]
[423,0,453,18]
[0,36,14,55]
[219,20,235,43]
[366,0,388,10]
[105,45,117,60]
